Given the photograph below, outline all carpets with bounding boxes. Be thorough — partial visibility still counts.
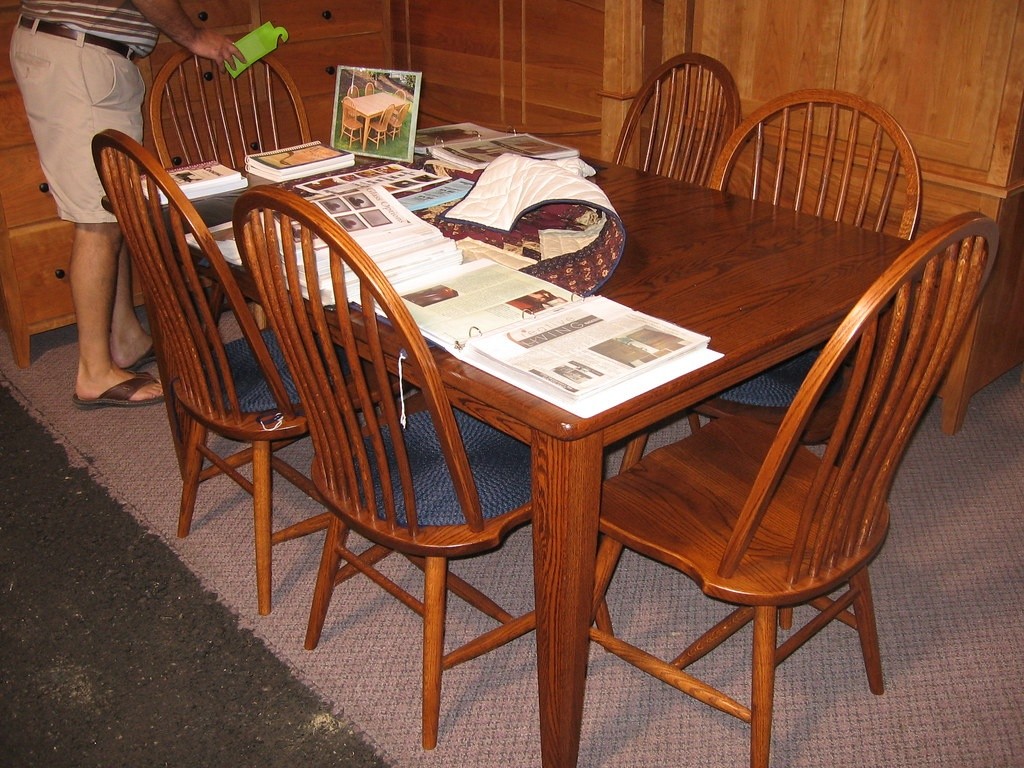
[1,300,1024,768]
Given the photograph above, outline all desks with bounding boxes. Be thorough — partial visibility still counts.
[100,142,948,768]
[341,93,408,152]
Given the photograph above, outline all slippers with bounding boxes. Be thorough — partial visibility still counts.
[120,340,157,372]
[72,370,165,410]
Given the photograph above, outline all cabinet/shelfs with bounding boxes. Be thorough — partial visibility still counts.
[0,0,392,369]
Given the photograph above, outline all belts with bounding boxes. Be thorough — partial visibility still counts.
[20,17,136,62]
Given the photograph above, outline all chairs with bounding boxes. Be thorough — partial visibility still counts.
[89,41,616,749]
[583,50,1000,768]
[339,82,410,149]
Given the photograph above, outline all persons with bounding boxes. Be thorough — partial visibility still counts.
[8,0,247,408]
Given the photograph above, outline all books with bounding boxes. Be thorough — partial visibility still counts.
[131,122,724,418]
